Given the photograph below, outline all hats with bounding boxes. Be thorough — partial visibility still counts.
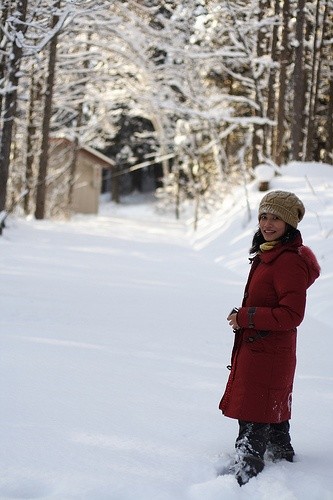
[259,191,305,228]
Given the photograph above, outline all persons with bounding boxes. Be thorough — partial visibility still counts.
[219,191,321,487]
